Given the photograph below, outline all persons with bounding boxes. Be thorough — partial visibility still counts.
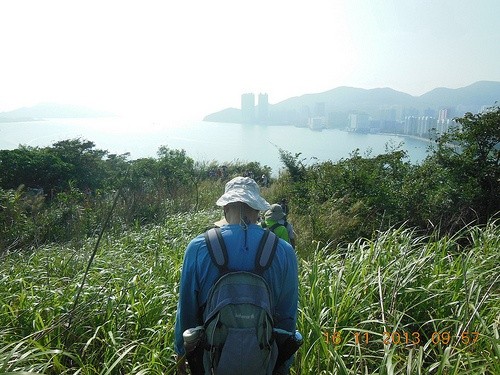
[174,175,306,374]
[256,197,296,250]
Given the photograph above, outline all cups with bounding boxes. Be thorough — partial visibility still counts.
[182,326,205,375]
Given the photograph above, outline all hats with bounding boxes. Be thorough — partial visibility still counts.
[263,204,286,220]
[217,176,270,210]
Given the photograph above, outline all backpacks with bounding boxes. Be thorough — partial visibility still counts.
[183,226,304,374]
